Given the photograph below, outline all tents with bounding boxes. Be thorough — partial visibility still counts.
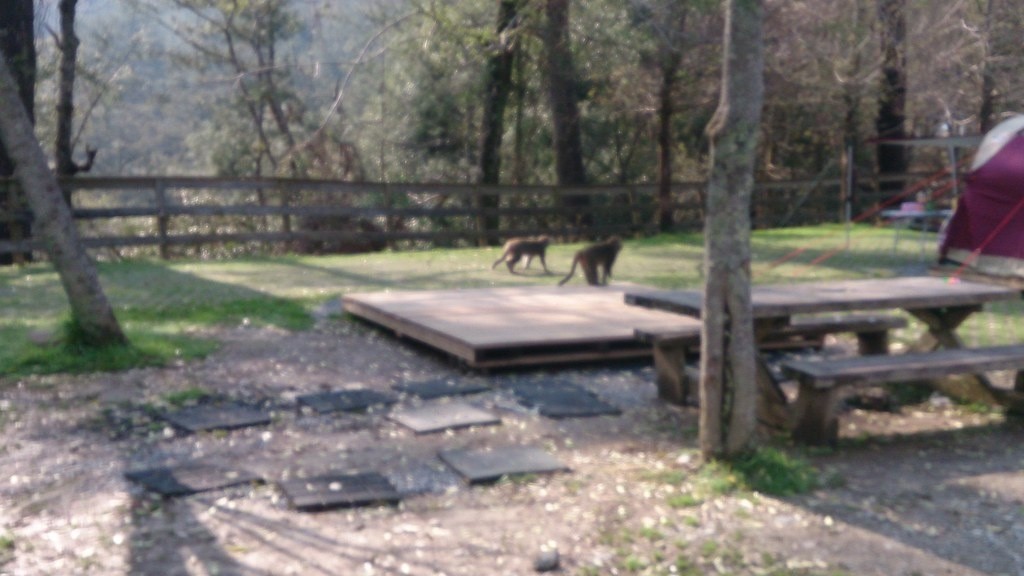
[935,111,1024,281]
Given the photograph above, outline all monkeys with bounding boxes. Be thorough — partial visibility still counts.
[559,236,623,287]
[492,236,551,274]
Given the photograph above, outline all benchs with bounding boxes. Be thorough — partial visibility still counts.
[633,318,909,407]
[778,343,1024,444]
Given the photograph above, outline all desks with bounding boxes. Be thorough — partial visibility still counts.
[881,208,953,266]
[623,272,1024,447]
